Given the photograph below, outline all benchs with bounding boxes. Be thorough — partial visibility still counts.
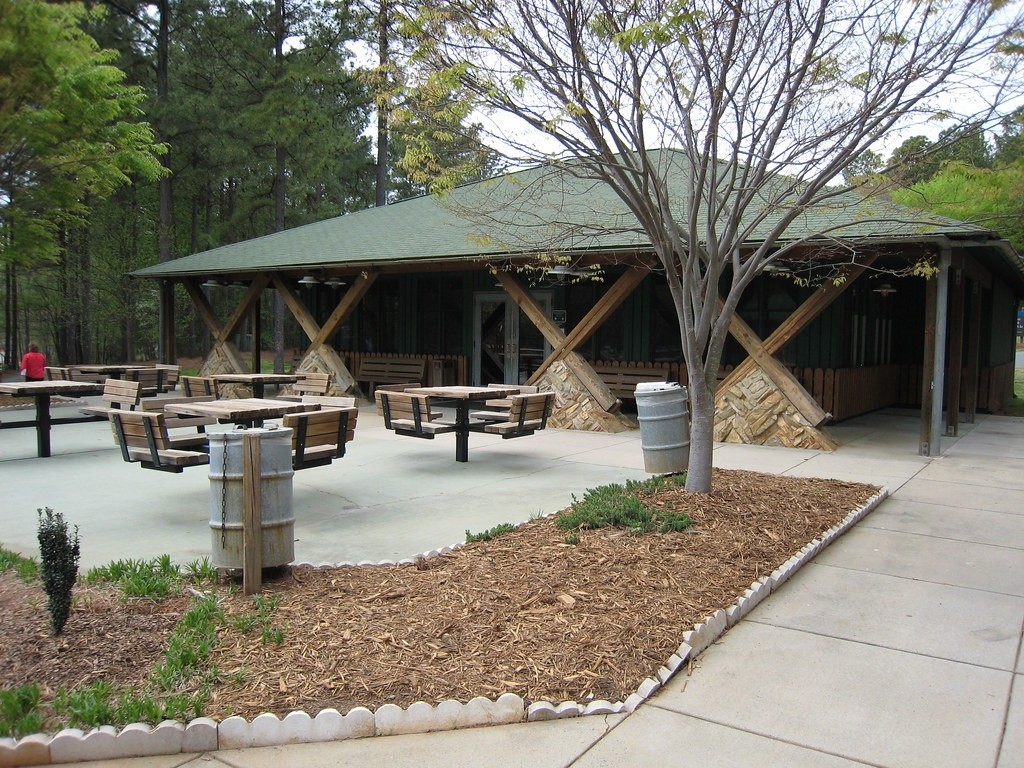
[179,375,219,401]
[290,356,349,372]
[156,364,182,392]
[355,357,427,397]
[485,392,556,439]
[470,384,539,422]
[108,409,208,472]
[376,384,443,419]
[275,372,331,402]
[284,406,357,470]
[79,379,143,418]
[125,368,167,397]
[302,395,358,412]
[375,390,452,438]
[44,366,72,381]
[140,396,216,447]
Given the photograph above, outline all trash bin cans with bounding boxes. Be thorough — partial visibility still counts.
[633,381,690,474]
[206,420,297,569]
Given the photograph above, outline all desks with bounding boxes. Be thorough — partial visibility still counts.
[0,380,96,458]
[75,365,155,409]
[164,398,321,429]
[404,386,520,462]
[592,366,670,398]
[212,373,306,427]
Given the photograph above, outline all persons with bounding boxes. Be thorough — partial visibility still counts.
[18,342,47,382]
